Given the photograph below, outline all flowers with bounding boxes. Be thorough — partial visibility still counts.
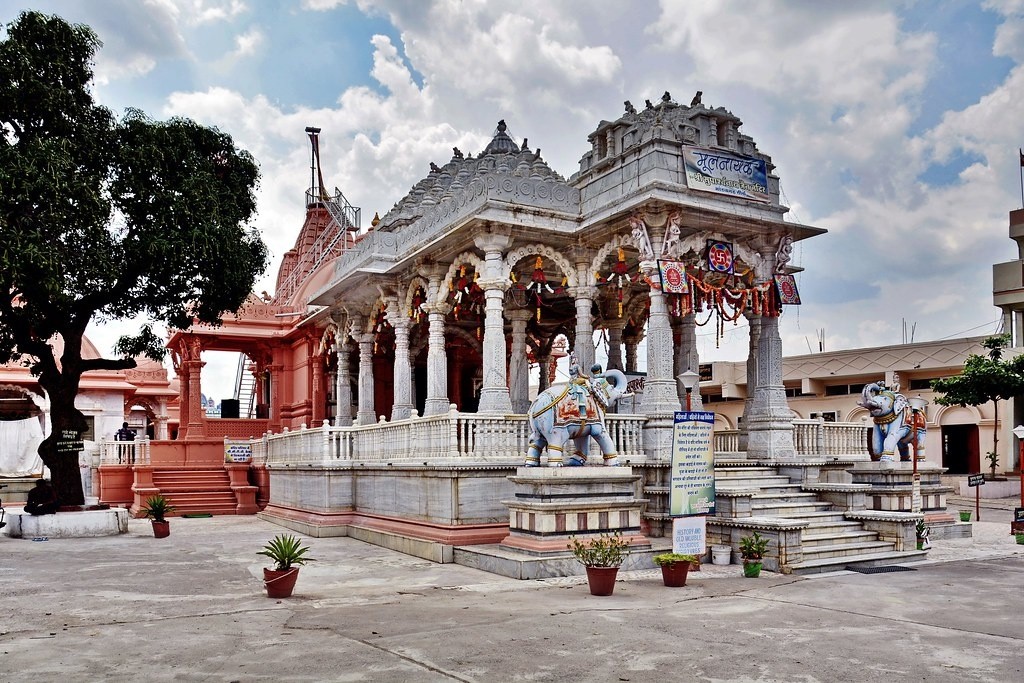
[739,532,771,568]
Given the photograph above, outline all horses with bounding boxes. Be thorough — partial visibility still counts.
[261,291,272,302]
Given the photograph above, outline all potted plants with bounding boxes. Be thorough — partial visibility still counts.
[139,493,178,539]
[915,518,930,550]
[255,533,312,598]
[568,529,634,596]
[652,552,699,588]
[1012,523,1024,544]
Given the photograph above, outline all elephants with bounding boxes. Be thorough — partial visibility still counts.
[524,369,628,467]
[857,382,927,463]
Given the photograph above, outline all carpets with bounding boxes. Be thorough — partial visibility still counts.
[846,566,918,574]
[181,514,213,518]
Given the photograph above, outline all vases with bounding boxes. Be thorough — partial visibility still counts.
[960,510,971,521]
[740,557,763,578]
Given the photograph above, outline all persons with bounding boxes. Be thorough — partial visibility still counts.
[24,479,59,516]
[114,422,136,464]
